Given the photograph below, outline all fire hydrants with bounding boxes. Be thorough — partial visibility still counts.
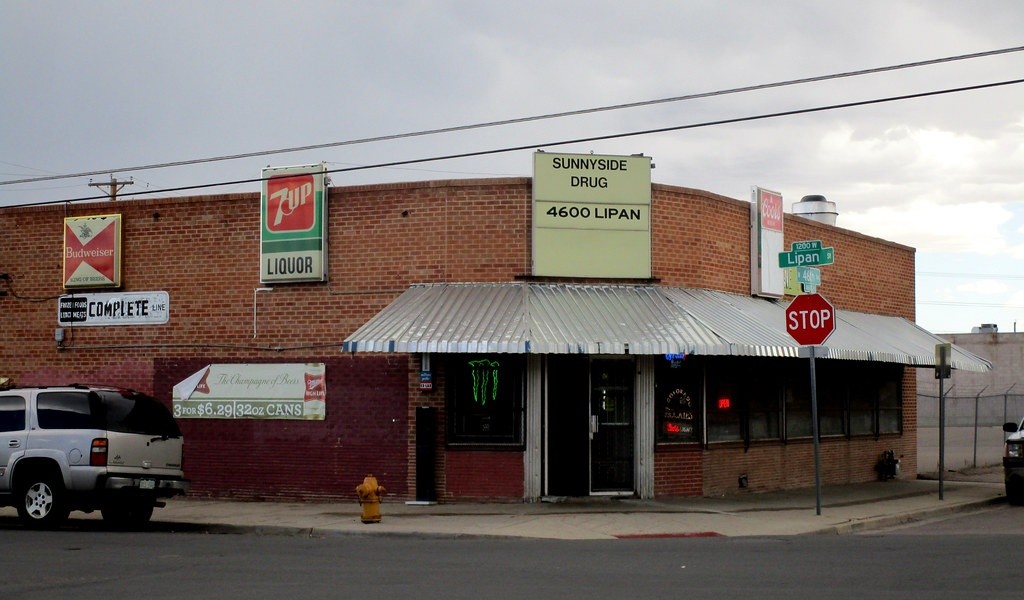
[356,473,386,523]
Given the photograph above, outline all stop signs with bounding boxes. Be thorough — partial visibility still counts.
[785,294,836,347]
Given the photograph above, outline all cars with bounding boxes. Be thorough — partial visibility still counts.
[0,383,191,530]
[1002,416,1024,506]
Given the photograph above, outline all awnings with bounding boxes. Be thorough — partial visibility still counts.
[339,282,994,372]
